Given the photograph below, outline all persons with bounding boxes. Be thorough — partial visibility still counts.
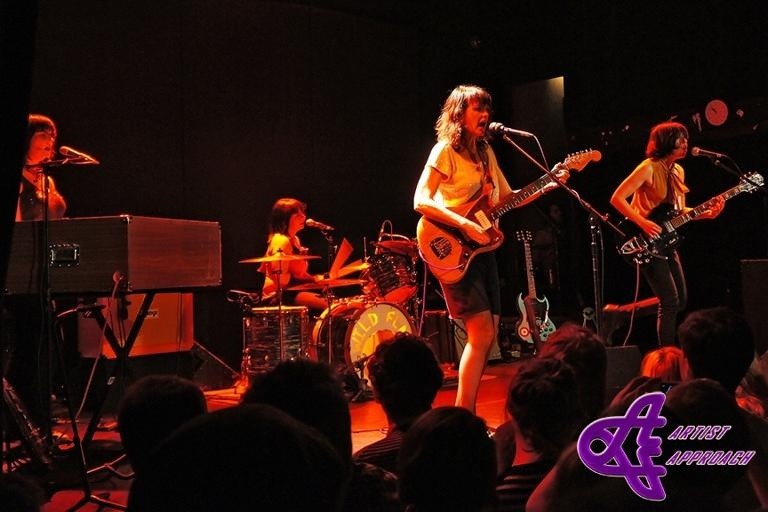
[528,202,589,313]
[113,303,767,511]
[609,120,726,348]
[411,82,570,417]
[256,193,347,313]
[4,111,72,449]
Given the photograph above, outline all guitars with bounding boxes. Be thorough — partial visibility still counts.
[515,228,558,344]
[609,170,764,266]
[416,147,601,285]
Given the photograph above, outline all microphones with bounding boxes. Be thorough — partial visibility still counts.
[488,122,535,138]
[691,146,726,158]
[306,218,327,229]
[58,144,98,163]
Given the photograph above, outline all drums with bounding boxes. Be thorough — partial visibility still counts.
[249,305,313,374]
[312,298,418,391]
[357,254,420,305]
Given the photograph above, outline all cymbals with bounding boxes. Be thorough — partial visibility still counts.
[285,276,366,289]
[238,249,323,264]
[370,238,422,255]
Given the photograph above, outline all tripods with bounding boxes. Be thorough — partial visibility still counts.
[5,172,95,503]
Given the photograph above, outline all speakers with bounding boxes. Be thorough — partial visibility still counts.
[605,346,641,388]
[740,259,767,352]
